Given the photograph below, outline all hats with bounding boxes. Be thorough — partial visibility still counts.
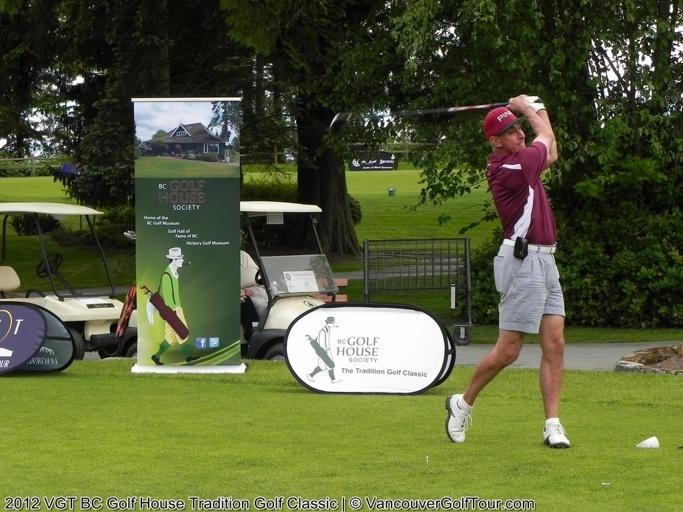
[483,107,526,141]
[165,247,184,259]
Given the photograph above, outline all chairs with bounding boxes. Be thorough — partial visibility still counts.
[0,267,19,298]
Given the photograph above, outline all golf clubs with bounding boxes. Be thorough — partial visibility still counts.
[140,284,153,295]
[306,335,313,341]
[330,97,543,137]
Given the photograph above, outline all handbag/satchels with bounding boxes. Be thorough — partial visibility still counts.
[513,237,528,259]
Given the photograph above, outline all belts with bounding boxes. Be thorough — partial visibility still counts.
[503,239,557,254]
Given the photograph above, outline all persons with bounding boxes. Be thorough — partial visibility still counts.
[239,230,268,358]
[144,247,200,366]
[305,315,343,386]
[442,94,572,449]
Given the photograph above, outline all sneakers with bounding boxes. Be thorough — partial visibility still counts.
[542,421,571,448]
[445,393,472,443]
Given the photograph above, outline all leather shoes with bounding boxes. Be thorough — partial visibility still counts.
[150,354,163,365]
[186,355,199,362]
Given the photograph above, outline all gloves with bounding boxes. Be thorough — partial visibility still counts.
[508,94,546,113]
